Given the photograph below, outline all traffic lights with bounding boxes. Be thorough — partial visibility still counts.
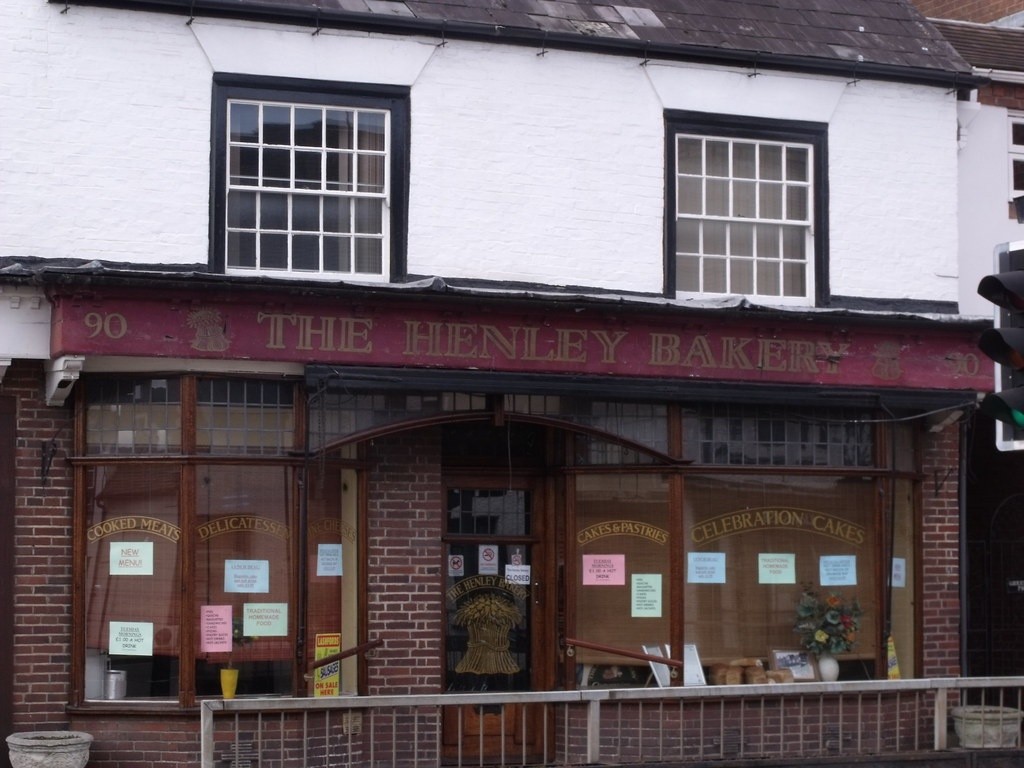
[976,270,1024,451]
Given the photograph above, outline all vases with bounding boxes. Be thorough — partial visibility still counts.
[951,704,1024,748]
[818,650,841,681]
[6,730,94,768]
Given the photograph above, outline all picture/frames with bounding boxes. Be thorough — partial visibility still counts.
[766,646,821,683]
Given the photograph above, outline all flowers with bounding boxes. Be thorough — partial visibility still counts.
[794,583,865,655]
